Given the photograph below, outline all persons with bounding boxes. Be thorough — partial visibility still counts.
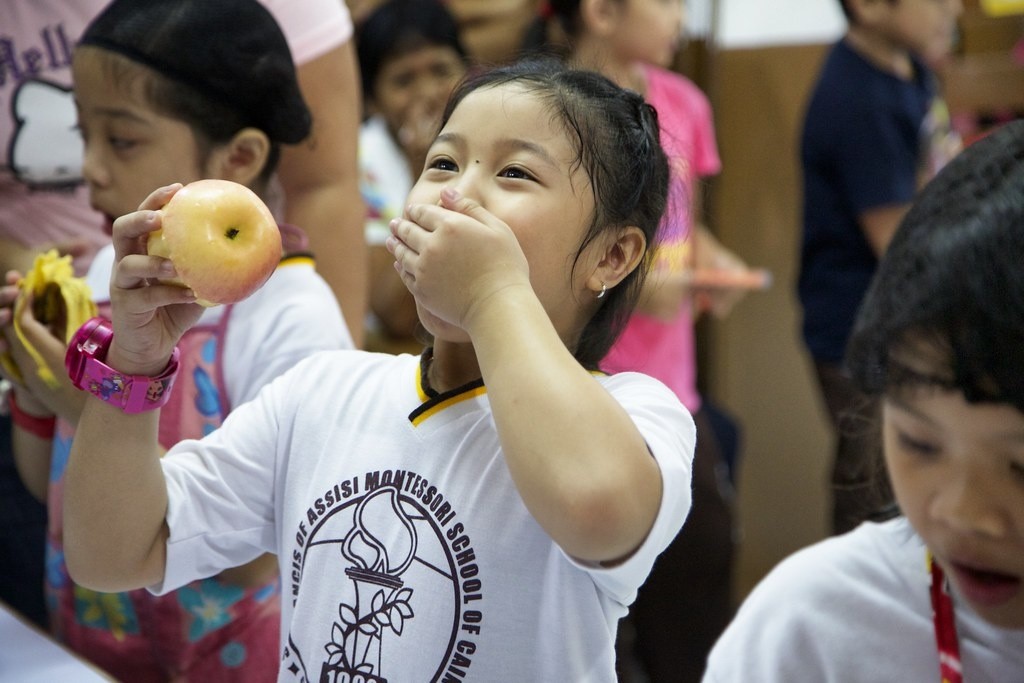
[0,1,357,683]
[0,0,369,628]
[701,119,1024,683]
[795,0,964,537]
[66,60,697,683]
[355,0,470,212]
[522,1,769,682]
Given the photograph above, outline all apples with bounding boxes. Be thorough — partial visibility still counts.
[146,179,283,310]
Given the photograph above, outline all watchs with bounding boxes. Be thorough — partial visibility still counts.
[10,396,55,439]
[65,316,180,415]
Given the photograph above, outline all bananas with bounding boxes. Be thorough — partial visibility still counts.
[0,249,99,392]
